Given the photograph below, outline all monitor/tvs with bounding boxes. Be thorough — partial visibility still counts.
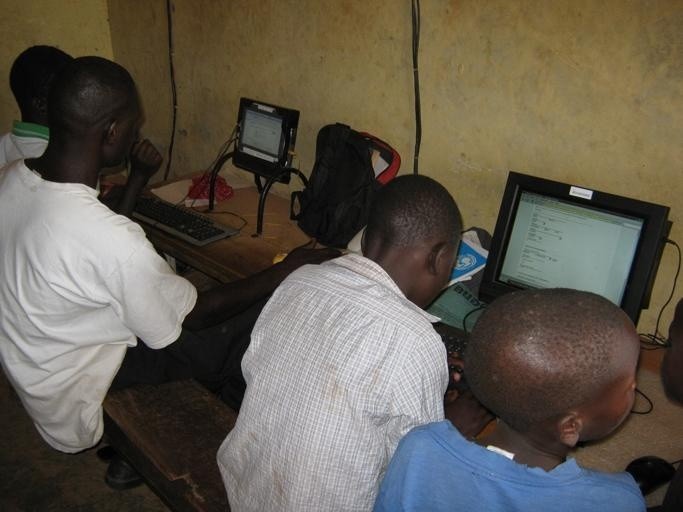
[230,97,299,185]
[474,166,675,338]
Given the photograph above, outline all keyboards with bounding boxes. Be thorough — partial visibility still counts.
[131,193,240,247]
[435,323,471,393]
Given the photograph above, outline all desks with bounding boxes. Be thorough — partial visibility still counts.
[129,168,683,512]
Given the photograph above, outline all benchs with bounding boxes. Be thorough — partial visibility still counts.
[106,371,239,512]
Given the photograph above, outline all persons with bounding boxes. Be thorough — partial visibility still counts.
[372,289,646,512]
[661,297,683,512]
[217,174,463,512]
[0,56,343,490]
[0,45,163,218]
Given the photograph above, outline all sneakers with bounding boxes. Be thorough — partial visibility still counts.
[104,456,145,490]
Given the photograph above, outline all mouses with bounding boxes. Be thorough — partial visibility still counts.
[625,455,677,496]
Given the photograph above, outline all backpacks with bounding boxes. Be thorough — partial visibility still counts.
[289,122,400,250]
